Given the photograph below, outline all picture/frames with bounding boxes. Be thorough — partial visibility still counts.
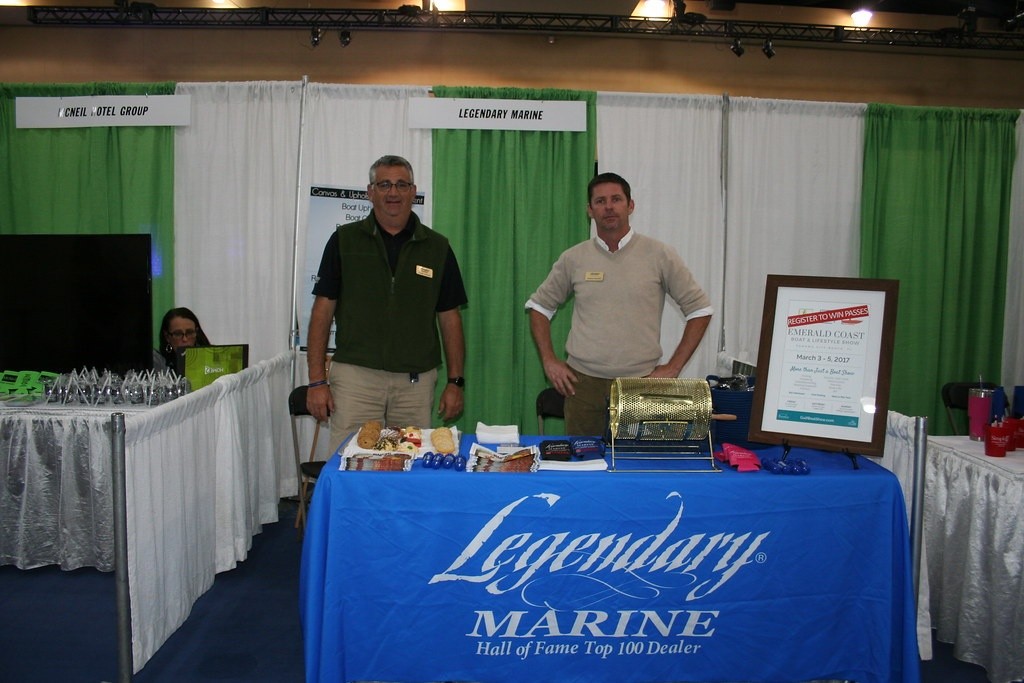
[749,274,901,457]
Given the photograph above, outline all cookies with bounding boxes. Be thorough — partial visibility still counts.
[431,426,455,453]
[357,421,381,449]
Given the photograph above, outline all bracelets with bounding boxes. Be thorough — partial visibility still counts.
[308,380,328,388]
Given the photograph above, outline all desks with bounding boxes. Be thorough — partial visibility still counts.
[923,434,1022,675]
[1,377,192,569]
[296,429,921,682]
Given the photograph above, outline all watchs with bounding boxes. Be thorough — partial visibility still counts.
[447,376,465,387]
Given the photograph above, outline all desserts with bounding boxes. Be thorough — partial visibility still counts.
[374,425,420,454]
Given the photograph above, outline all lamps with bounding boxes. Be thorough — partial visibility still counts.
[851,8,875,30]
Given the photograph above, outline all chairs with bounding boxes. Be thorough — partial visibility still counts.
[287,383,332,530]
[537,384,567,440]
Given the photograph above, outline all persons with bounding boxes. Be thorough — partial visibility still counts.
[308,154,469,463]
[523,171,715,439]
[160,307,210,378]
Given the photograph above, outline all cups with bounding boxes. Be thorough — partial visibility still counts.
[966,387,996,443]
